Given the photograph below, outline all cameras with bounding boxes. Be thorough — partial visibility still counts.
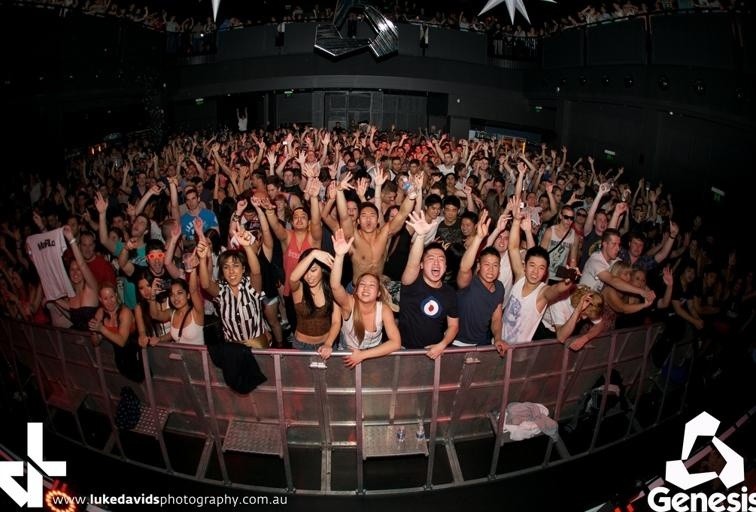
[156,279,172,290]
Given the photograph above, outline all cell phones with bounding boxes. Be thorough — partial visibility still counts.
[555,265,581,283]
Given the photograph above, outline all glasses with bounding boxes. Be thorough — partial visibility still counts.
[146,252,165,261]
[576,211,587,218]
[562,214,575,221]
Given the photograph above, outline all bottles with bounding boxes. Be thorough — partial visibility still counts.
[402,175,419,201]
[396,426,407,452]
[413,425,427,451]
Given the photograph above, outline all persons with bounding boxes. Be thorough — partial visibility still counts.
[235,105,248,132]
[14,0,751,38]
[0,117,756,448]
